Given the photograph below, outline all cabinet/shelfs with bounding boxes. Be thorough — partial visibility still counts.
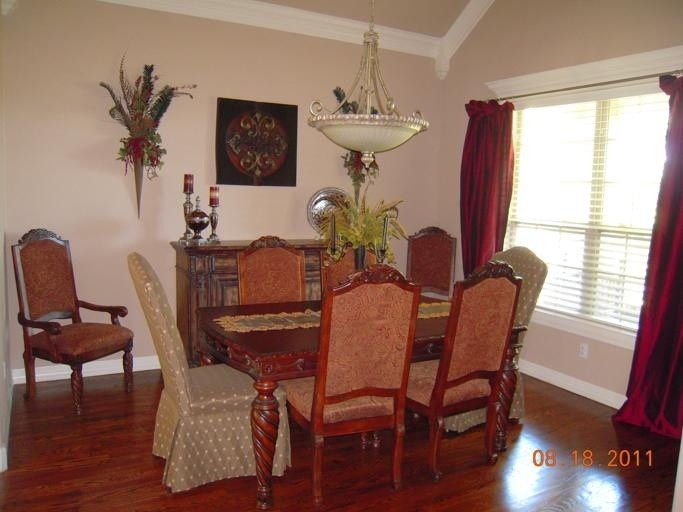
[168,236,352,369]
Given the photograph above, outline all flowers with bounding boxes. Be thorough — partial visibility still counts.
[332,86,380,184]
[93,50,196,182]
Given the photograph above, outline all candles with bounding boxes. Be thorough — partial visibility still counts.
[184,173,193,192]
[210,186,218,205]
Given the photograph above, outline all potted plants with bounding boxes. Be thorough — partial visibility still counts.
[318,182,408,273]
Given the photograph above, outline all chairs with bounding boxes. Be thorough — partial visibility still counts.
[128,252,292,491]
[405,224,457,362]
[236,236,319,389]
[321,243,376,302]
[402,259,523,478]
[10,227,132,415]
[281,265,420,506]
[423,247,548,436]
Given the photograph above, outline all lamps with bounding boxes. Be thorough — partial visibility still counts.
[306,0,430,169]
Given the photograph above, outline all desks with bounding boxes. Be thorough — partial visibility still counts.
[194,292,529,512]
